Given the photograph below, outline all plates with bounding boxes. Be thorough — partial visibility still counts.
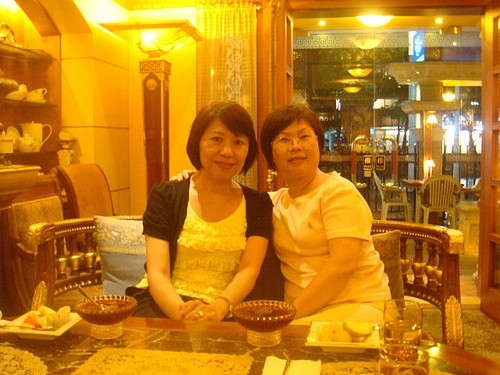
[6,126,21,151]
[0,309,82,341]
[0,22,16,46]
[304,320,381,354]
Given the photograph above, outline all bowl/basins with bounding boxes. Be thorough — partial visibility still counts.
[231,300,297,348]
[75,295,137,340]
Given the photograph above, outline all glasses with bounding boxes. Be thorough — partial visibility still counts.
[272,133,319,148]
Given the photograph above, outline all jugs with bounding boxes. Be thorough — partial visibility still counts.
[19,121,52,152]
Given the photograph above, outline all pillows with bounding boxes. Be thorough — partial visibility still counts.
[371,230,405,321]
[95,217,146,296]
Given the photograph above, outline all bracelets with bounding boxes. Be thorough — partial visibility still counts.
[215,296,234,319]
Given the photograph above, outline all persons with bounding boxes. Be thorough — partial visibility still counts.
[170,103,401,328]
[129,98,274,320]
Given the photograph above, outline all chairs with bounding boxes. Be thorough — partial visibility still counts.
[58,163,115,220]
[372,171,461,229]
[0,181,63,314]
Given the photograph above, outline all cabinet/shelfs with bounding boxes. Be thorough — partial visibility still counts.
[0,0,61,174]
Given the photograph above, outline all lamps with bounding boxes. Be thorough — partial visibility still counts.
[347,67,373,78]
[99,20,205,202]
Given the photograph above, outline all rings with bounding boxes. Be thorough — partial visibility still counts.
[197,310,204,318]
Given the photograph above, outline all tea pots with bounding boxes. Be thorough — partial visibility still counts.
[13,132,36,153]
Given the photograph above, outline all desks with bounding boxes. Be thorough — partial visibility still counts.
[401,180,464,223]
[1,314,500,375]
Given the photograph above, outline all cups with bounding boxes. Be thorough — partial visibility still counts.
[5,91,27,101]
[378,344,430,375]
[24,88,47,102]
[0,138,14,153]
[382,299,423,363]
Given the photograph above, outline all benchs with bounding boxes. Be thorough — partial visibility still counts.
[38,215,465,349]
